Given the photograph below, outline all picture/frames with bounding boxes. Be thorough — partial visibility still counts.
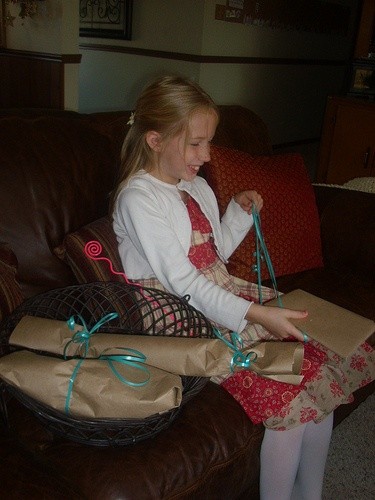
[78,0,132,41]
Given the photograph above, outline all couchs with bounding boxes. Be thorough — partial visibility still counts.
[1,97,374,500]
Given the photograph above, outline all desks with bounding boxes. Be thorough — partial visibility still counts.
[0,47,64,108]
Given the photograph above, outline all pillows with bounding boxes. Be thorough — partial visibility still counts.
[196,141,326,286]
[56,212,141,335]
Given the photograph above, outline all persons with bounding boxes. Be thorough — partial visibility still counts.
[107,71,375,500]
